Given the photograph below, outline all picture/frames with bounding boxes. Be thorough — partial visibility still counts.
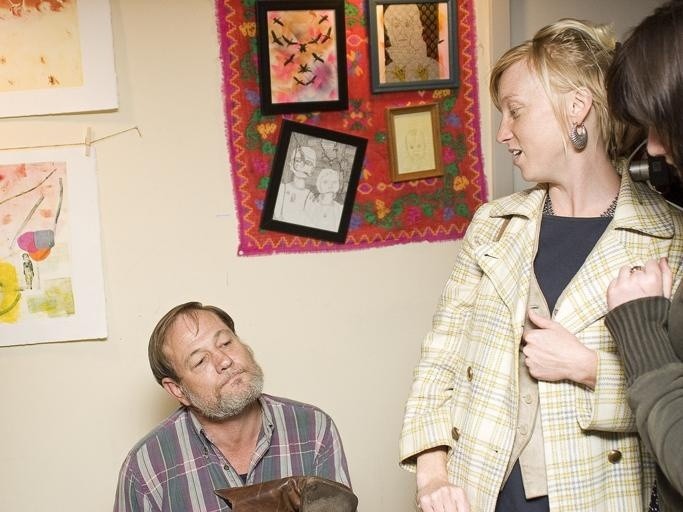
[0,145,108,347]
[259,120,368,244]
[385,102,445,183]
[0,0,119,118]
[255,0,349,115]
[368,0,460,93]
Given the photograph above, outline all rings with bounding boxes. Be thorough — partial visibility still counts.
[630,266,643,273]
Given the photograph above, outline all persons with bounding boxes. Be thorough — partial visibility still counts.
[603,1,683,512]
[112,302,359,512]
[398,17,683,512]
[273,146,344,232]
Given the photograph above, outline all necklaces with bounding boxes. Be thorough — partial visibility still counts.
[542,194,619,218]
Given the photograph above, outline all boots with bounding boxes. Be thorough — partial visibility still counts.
[214,475,358,512]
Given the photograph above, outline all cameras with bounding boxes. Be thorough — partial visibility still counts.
[629,152,676,193]
